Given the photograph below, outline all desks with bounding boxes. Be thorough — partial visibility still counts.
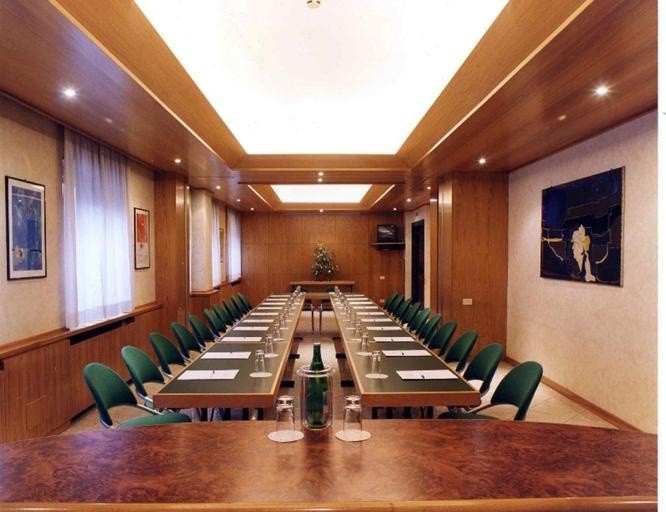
[330,292,482,418]
[290,280,355,293]
[155,293,307,421]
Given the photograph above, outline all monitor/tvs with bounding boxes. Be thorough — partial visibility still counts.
[376,224,400,241]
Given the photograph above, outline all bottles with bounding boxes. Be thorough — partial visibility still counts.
[305,343,327,428]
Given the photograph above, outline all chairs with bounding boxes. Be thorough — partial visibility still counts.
[378,291,542,422]
[83,292,253,431]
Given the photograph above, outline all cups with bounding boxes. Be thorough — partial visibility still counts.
[342,395,362,438]
[335,286,383,377]
[255,286,301,374]
[275,395,295,439]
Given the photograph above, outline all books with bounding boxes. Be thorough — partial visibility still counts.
[177,294,292,380]
[344,295,459,380]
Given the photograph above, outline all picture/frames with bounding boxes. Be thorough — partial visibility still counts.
[134,208,150,269]
[5,175,47,280]
